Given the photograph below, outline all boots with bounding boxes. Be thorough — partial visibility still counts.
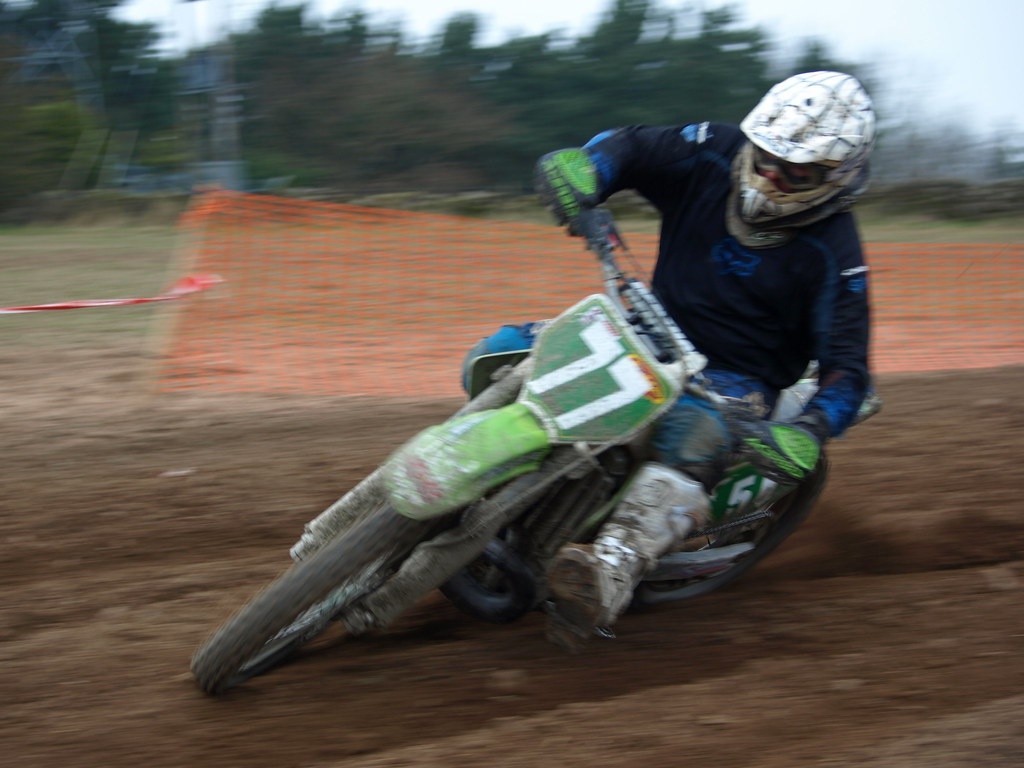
[544,460,715,657]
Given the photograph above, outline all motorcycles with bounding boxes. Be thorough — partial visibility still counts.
[188,206,885,701]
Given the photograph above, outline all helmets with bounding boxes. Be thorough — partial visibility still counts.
[735,70,876,229]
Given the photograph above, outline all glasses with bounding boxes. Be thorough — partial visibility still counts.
[746,139,828,194]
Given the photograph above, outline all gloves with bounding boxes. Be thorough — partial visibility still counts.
[534,146,604,228]
[741,419,823,487]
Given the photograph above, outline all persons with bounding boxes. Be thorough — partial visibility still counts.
[459,69,877,658]
